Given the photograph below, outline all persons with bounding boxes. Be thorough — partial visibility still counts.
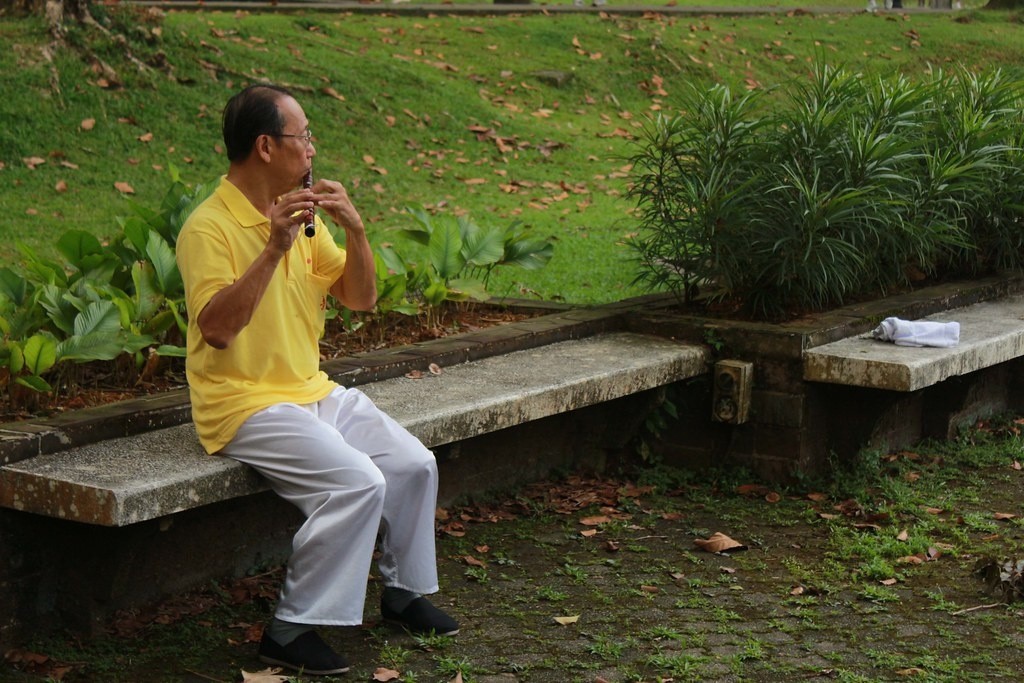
[175,85,461,675]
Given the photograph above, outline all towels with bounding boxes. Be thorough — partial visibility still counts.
[872,315,961,349]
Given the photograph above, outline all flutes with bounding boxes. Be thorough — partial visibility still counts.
[303,169,316,237]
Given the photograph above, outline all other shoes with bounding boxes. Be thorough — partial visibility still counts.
[380,596,460,637]
[258,627,349,675]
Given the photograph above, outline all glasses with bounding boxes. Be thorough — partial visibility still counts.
[256,130,312,145]
